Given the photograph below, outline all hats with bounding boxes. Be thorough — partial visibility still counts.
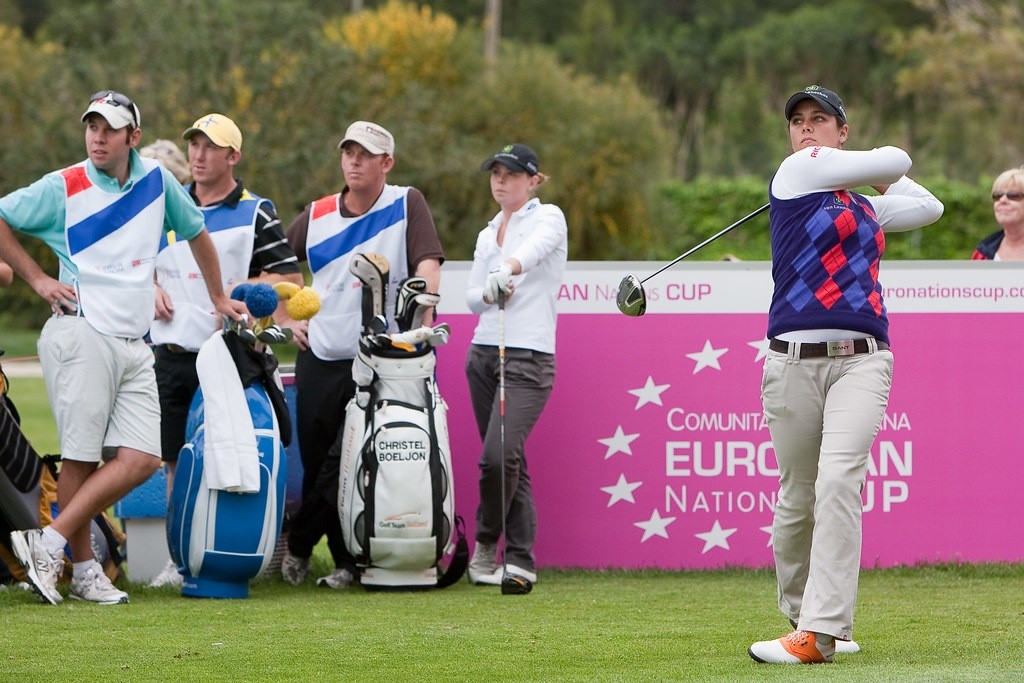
[481,143,539,176]
[181,113,243,151]
[785,85,848,124]
[80,90,141,129]
[338,121,395,156]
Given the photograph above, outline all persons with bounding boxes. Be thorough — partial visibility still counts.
[0,90,313,605]
[973,165,1024,260]
[465,143,568,586]
[747,85,944,664]
[261,121,446,589]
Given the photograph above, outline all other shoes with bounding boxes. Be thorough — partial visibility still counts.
[147,558,184,589]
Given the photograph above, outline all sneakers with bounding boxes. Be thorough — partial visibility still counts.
[747,631,836,664]
[68,562,130,605]
[475,564,537,584]
[789,618,860,653]
[282,551,309,586]
[10,528,65,606]
[317,568,353,589]
[467,540,497,584]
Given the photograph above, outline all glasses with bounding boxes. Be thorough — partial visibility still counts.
[992,191,1024,201]
[87,91,138,127]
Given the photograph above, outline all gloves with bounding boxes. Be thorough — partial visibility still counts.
[486,263,512,303]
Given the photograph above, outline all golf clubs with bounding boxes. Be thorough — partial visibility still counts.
[367,314,452,351]
[496,287,535,596]
[616,201,771,317]
[209,310,295,355]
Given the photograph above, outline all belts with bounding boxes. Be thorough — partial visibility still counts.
[769,336,890,356]
[52,304,84,317]
